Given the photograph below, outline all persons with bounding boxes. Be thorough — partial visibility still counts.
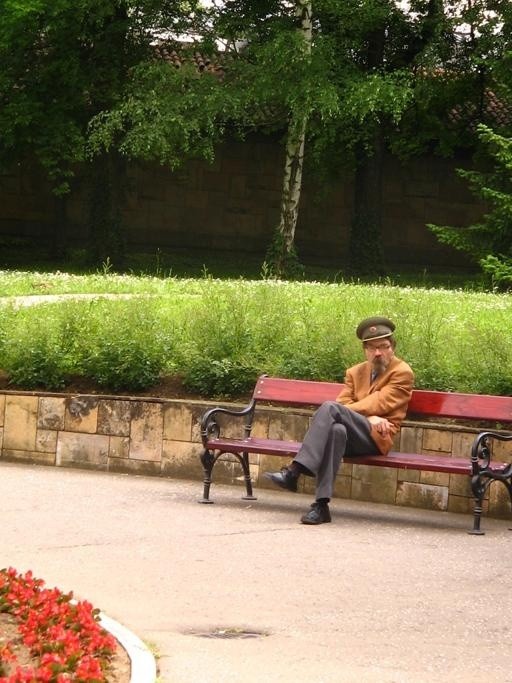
[265,317,414,525]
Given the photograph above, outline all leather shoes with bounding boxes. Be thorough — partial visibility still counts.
[262,468,297,491]
[301,505,331,525]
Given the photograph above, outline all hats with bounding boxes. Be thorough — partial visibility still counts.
[356,317,396,341]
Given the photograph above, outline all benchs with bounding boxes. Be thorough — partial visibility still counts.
[197,371,511,537]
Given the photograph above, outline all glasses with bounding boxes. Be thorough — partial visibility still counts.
[364,344,392,351]
